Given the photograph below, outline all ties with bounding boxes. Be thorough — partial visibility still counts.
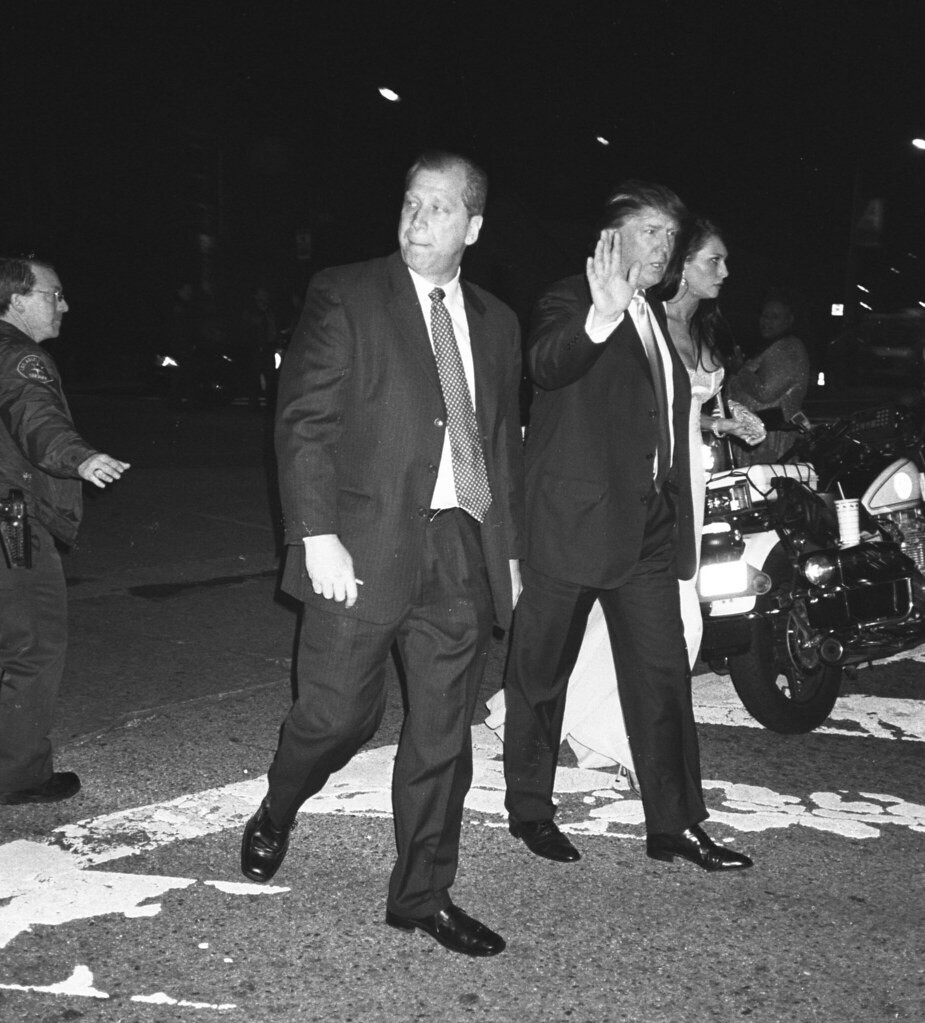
[632,287,672,493]
[428,287,492,524]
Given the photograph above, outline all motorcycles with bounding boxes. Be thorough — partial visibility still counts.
[697,342,924,737]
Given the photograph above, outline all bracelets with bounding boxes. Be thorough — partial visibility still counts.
[712,418,726,437]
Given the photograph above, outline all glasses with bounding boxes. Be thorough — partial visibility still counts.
[18,289,65,302]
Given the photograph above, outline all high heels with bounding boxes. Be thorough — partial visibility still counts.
[615,763,641,796]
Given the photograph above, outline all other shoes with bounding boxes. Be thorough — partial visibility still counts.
[0,770,80,806]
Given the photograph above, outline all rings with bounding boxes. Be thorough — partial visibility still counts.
[94,468,102,477]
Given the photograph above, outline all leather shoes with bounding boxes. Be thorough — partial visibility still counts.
[386,903,506,957]
[508,820,580,862]
[240,797,298,882]
[646,824,754,872]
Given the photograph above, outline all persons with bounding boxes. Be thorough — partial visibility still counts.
[483,218,767,791]
[242,148,524,957]
[0,249,131,805]
[505,181,755,872]
[720,298,810,468]
